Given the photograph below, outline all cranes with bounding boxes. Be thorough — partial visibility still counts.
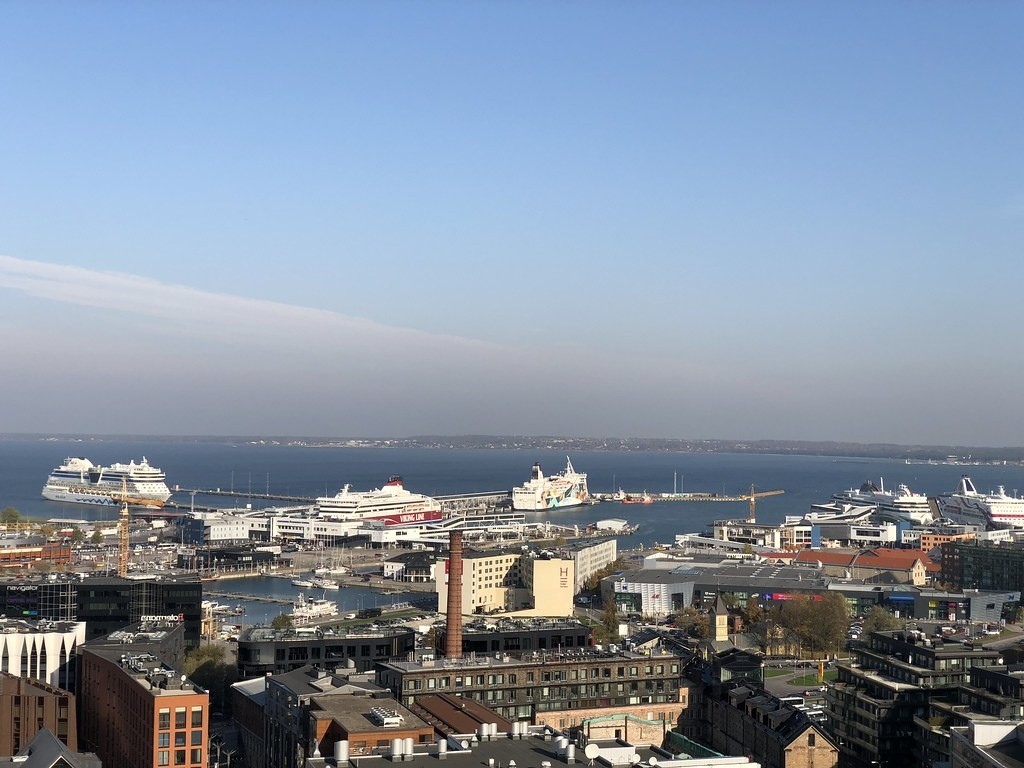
[112,479,166,575]
[740,483,784,519]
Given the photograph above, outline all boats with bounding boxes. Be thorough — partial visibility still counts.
[202,600,240,642]
[41,455,173,512]
[513,456,589,511]
[622,497,654,504]
[784,476,1024,534]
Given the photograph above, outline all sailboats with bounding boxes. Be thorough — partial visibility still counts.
[263,535,353,618]
